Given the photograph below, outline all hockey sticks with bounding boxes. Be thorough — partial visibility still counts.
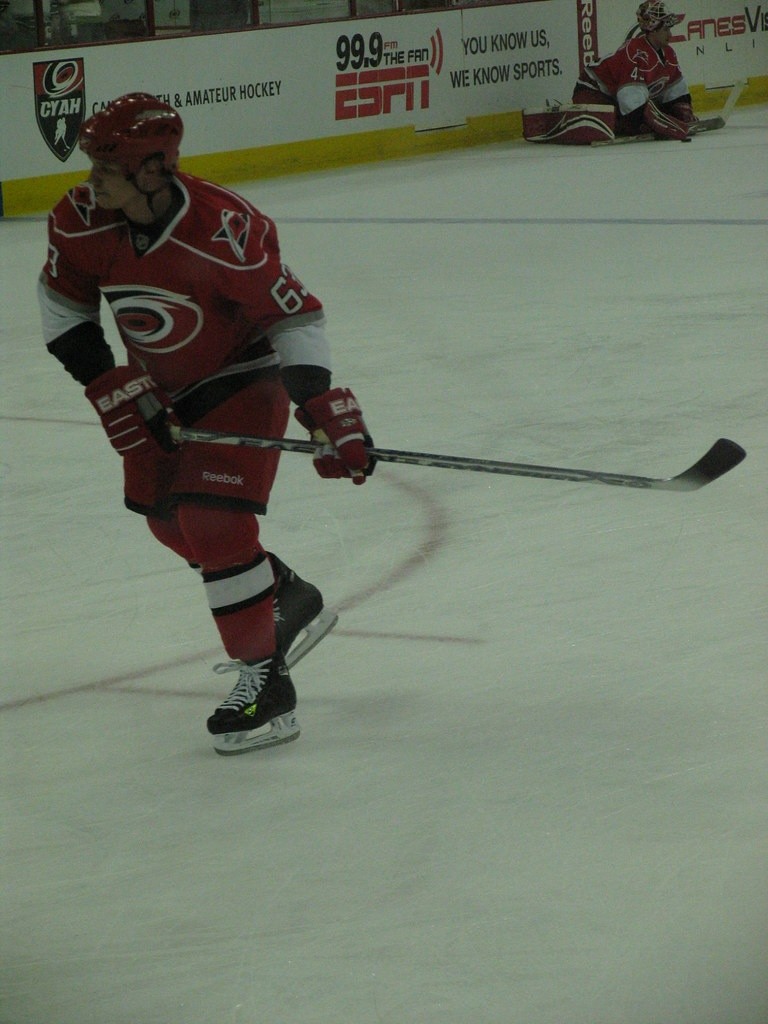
[178,420,749,495]
[589,76,746,149]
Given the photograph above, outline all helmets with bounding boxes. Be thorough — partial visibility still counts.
[81,92,183,181]
[636,0,685,33]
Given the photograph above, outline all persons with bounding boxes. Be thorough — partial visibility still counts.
[572,0,700,140]
[36,91,379,757]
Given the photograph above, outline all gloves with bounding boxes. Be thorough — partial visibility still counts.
[294,387,377,485]
[85,365,181,467]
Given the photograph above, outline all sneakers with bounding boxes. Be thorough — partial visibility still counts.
[207,653,299,756]
[266,551,338,667]
